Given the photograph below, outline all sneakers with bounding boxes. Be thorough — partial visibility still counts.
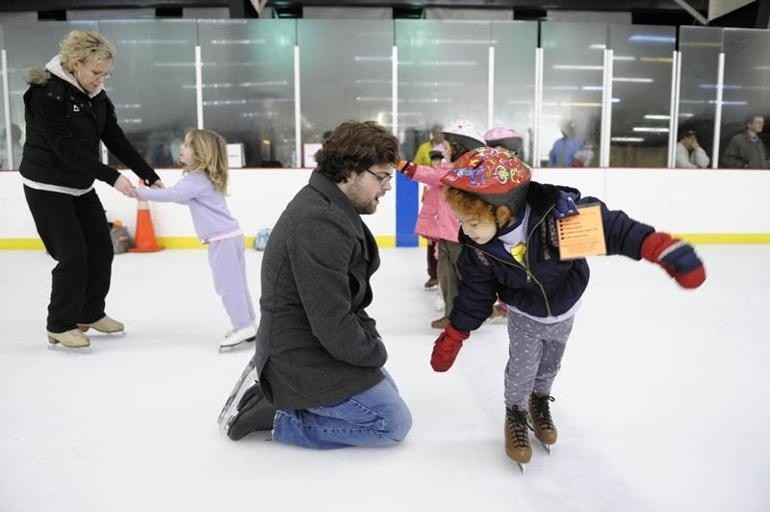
[226,393,276,440]
[425,278,438,286]
[48,329,90,347]
[504,409,531,463]
[220,325,258,346]
[77,315,124,332]
[530,392,557,443]
[432,317,450,328]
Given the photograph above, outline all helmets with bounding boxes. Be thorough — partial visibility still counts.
[440,147,532,215]
[484,127,522,146]
[441,122,487,150]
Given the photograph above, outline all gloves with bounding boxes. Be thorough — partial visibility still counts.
[640,233,704,288]
[397,160,416,178]
[431,317,469,371]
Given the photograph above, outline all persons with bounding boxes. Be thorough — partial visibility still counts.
[397,116,602,168]
[426,141,449,169]
[393,119,488,331]
[483,126,523,156]
[18,30,164,350]
[131,128,258,348]
[676,129,710,168]
[226,120,411,452]
[104,114,334,168]
[719,114,768,168]
[430,146,706,465]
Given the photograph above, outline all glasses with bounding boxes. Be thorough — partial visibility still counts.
[365,168,393,182]
[82,63,111,78]
